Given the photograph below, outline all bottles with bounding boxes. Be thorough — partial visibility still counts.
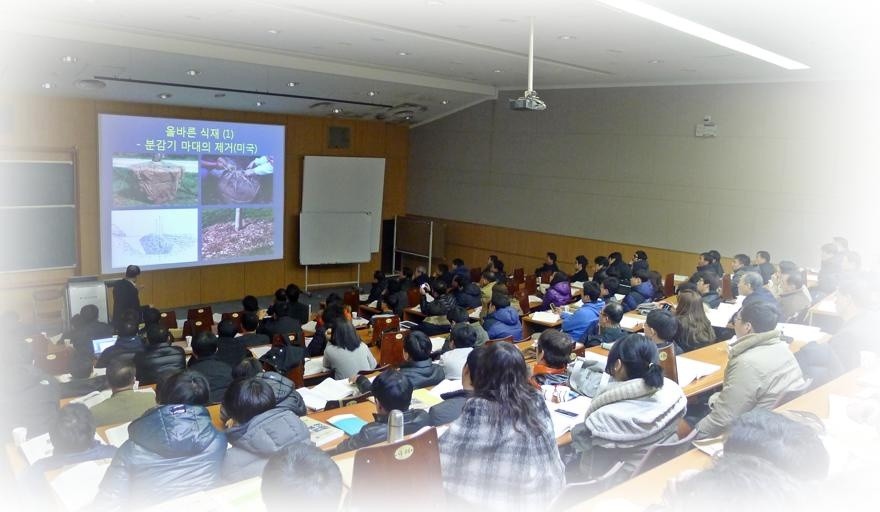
[384,408,404,443]
[367,324,373,337]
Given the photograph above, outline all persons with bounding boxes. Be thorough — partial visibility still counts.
[243,154,274,177]
[201,159,224,184]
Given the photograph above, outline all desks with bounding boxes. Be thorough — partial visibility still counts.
[3,264,864,512]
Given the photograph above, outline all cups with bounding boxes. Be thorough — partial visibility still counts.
[64,337,71,347]
[133,381,140,391]
[185,336,193,348]
[352,312,358,321]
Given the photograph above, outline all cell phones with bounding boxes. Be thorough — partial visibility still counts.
[550,303,557,312]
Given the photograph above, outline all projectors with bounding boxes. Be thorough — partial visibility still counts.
[510,99,547,111]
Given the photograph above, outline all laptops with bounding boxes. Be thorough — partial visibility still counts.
[91,335,119,360]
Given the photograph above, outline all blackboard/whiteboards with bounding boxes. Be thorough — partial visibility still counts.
[300,212,371,265]
